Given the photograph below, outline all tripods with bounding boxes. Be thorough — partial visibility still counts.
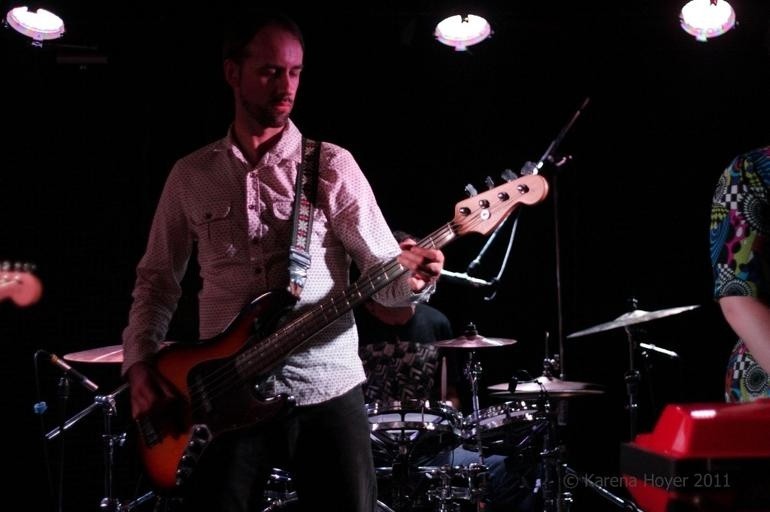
[489,97,645,512]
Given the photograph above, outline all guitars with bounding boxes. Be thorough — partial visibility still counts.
[127,155,552,497]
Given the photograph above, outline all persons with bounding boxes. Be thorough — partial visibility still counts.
[356,231,461,410]
[709,146,770,403]
[122,13,446,512]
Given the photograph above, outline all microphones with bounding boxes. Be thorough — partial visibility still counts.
[639,343,679,362]
[47,353,100,392]
[440,269,493,289]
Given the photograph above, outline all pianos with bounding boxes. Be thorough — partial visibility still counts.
[621,395,768,512]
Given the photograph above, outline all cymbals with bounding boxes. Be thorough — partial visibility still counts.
[565,306,702,340]
[64,339,176,365]
[488,377,605,400]
[428,337,516,348]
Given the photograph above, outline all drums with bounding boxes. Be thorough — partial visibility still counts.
[264,466,288,495]
[374,466,487,512]
[463,398,556,453]
[364,400,463,466]
[263,490,396,512]
